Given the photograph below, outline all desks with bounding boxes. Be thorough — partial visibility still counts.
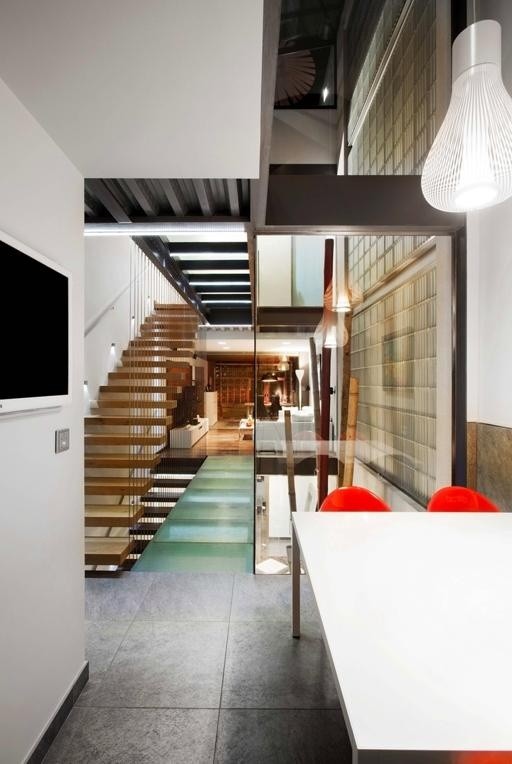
[291,512,512,764]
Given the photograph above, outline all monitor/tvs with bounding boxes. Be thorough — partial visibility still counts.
[0,231,72,413]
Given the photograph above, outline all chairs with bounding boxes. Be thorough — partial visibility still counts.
[252,395,267,421]
[427,486,501,512]
[269,395,282,421]
[317,486,392,512]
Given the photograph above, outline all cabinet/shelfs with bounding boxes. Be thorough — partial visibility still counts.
[170,418,209,449]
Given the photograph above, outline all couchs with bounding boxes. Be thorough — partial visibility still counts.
[253,406,332,455]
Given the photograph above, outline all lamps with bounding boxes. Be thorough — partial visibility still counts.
[277,352,289,370]
[420,0,512,214]
[313,236,364,349]
[295,370,305,411]
[261,374,278,382]
